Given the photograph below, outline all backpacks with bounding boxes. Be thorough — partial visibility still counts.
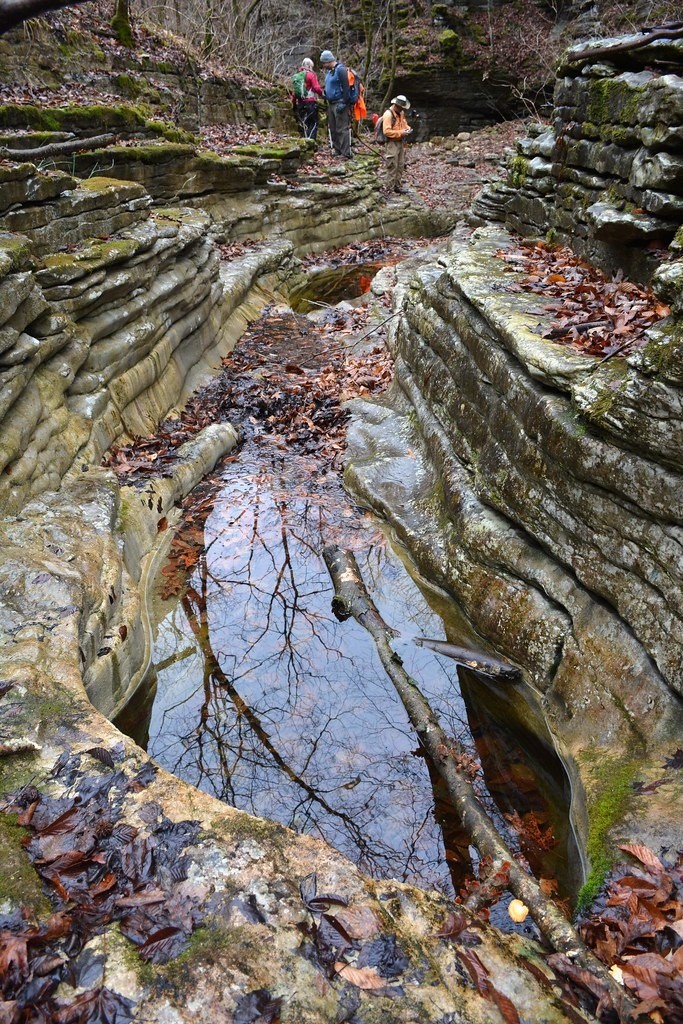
[337,64,360,105]
[374,108,396,144]
[292,71,314,99]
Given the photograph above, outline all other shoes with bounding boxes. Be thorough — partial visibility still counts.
[394,185,408,193]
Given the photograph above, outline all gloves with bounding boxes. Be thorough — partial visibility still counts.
[336,104,347,113]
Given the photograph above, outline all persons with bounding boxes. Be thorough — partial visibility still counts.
[291,58,322,141]
[319,49,359,158]
[382,95,413,193]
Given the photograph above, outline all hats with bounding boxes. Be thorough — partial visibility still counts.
[320,51,335,62]
[391,95,411,110]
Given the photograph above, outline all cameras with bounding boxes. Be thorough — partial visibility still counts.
[405,130,412,133]
